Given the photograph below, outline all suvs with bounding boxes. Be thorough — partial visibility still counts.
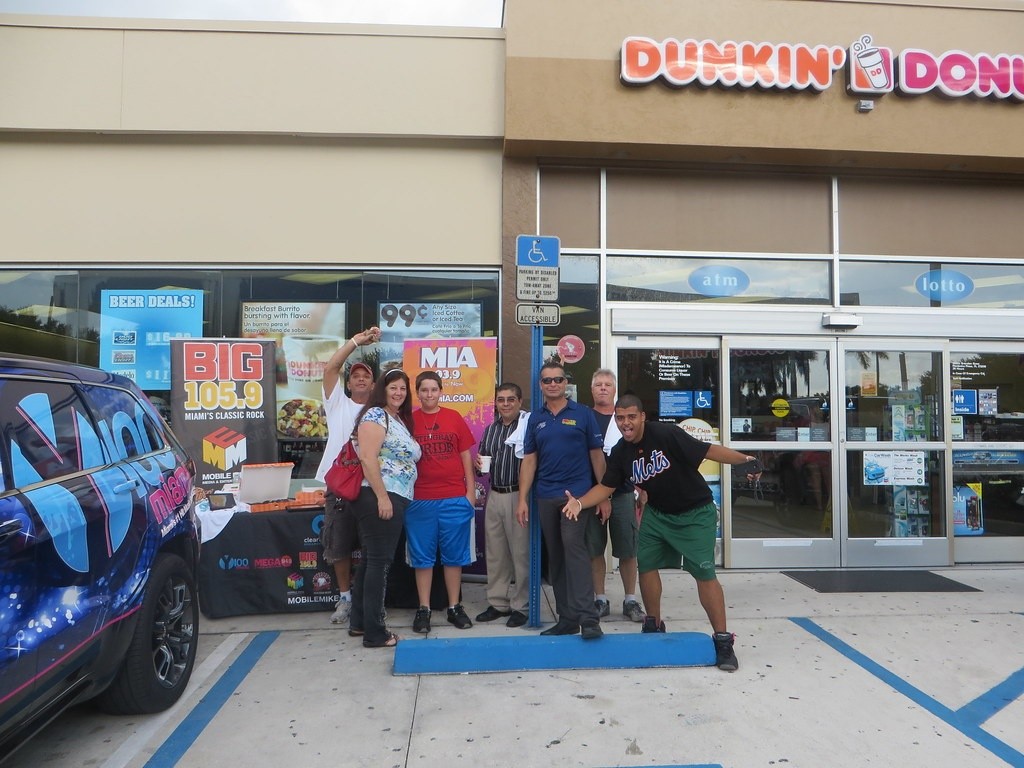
[0,353,201,768]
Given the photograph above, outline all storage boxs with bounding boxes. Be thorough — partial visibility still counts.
[238,462,326,512]
[882,482,983,539]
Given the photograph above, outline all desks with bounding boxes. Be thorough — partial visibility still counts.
[196,508,361,618]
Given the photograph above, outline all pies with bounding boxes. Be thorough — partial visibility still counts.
[276,388,328,437]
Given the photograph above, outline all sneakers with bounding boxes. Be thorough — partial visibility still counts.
[413,605,431,633]
[712,632,738,671]
[476,606,511,621]
[582,623,602,639]
[507,610,528,627]
[447,603,472,629]
[594,599,610,617]
[540,621,580,635]
[622,600,645,621]
[641,618,665,632]
[330,599,352,624]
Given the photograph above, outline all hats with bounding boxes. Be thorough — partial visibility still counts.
[350,362,373,376]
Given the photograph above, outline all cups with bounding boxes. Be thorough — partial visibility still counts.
[478,456,492,473]
[283,335,341,400]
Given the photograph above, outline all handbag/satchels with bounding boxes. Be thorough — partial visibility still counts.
[325,408,388,501]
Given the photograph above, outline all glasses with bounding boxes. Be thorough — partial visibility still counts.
[541,377,566,384]
[496,398,518,403]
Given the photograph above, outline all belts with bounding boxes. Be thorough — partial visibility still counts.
[491,485,519,493]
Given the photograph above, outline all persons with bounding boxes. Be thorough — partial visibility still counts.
[400,371,476,633]
[793,407,832,510]
[474,383,531,627]
[562,395,762,670]
[516,362,612,639]
[348,368,423,647]
[585,370,648,622]
[314,329,388,624]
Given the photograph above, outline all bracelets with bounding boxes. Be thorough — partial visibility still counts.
[351,338,358,347]
[745,456,753,462]
[577,500,582,511]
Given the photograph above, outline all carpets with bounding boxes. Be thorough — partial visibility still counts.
[781,569,984,593]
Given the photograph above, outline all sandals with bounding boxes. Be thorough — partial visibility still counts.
[363,633,400,647]
[349,627,364,636]
